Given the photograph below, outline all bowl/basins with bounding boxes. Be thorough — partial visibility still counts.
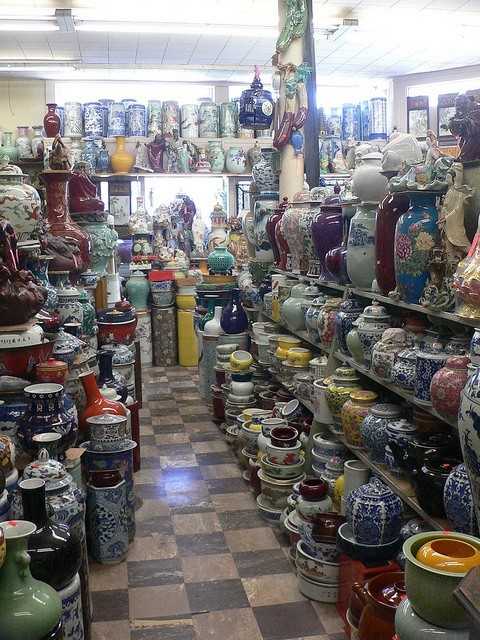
[241,411,274,481]
[222,370,257,428]
[280,477,333,547]
[310,433,346,476]
[252,321,272,365]
[256,426,304,523]
[210,344,238,418]
[268,334,312,383]
[230,350,254,370]
[296,510,347,604]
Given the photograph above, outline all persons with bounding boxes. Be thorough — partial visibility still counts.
[448,94,480,160]
[133,128,199,174]
[435,162,472,259]
[168,216,180,240]
[178,222,195,254]
[414,130,448,181]
[48,133,73,170]
[354,143,372,169]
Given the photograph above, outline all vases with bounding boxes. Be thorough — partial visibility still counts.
[177,310,199,366]
[85,478,129,565]
[0,132,16,161]
[83,102,104,137]
[259,392,275,410]
[295,540,340,603]
[393,189,444,304]
[312,378,334,424]
[220,286,243,334]
[272,274,286,320]
[43,103,60,138]
[256,426,306,523]
[208,247,235,274]
[79,440,137,545]
[251,322,312,382]
[296,495,332,523]
[311,205,342,282]
[299,202,323,279]
[149,280,175,308]
[327,106,342,137]
[176,283,197,310]
[81,139,97,174]
[182,104,198,138]
[240,419,284,490]
[282,201,309,275]
[107,102,126,138]
[272,402,286,420]
[301,478,328,502]
[396,599,470,640]
[310,431,354,480]
[77,371,127,432]
[32,433,62,462]
[282,398,310,424]
[15,126,30,158]
[199,298,217,331]
[35,171,92,272]
[374,169,409,298]
[151,307,177,366]
[27,255,57,313]
[111,136,133,174]
[220,104,235,138]
[64,102,83,139]
[208,225,229,250]
[285,511,306,563]
[225,146,246,174]
[54,574,85,640]
[251,147,280,194]
[341,460,370,499]
[347,201,382,293]
[252,200,277,262]
[226,408,267,444]
[210,344,256,422]
[161,101,181,137]
[1,521,62,639]
[18,478,82,592]
[205,140,225,173]
[128,105,146,137]
[200,103,219,138]
[117,240,133,264]
[204,306,224,337]
[31,124,43,157]
[17,383,79,461]
